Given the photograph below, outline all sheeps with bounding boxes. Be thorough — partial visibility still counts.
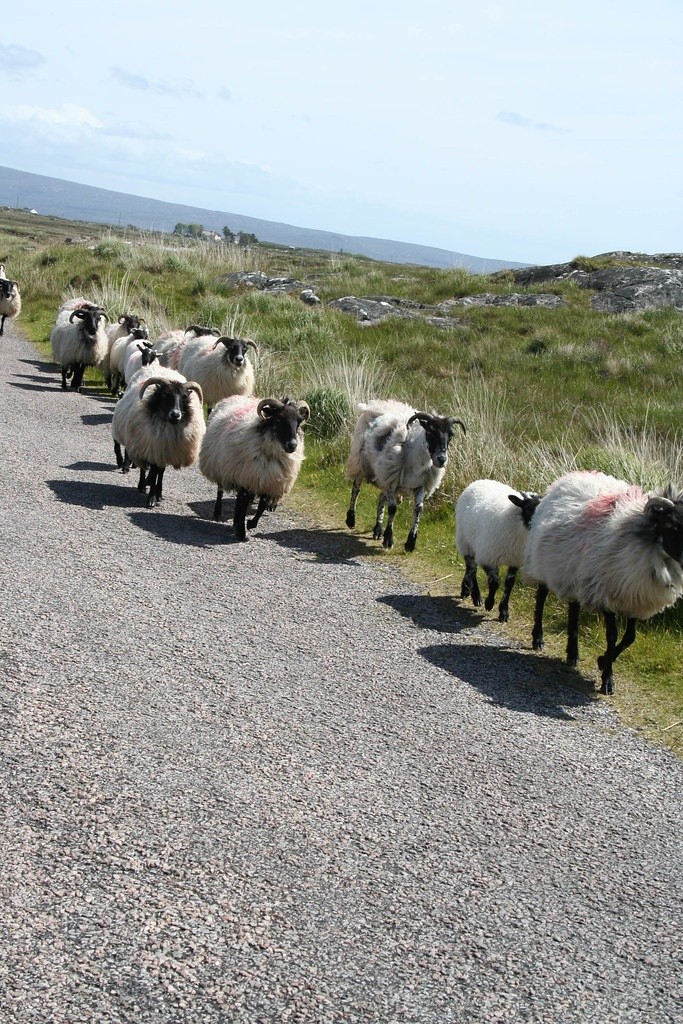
[521,468,683,696]
[454,479,544,624]
[0,262,22,337]
[344,399,467,553]
[199,394,311,540]
[50,294,260,510]
